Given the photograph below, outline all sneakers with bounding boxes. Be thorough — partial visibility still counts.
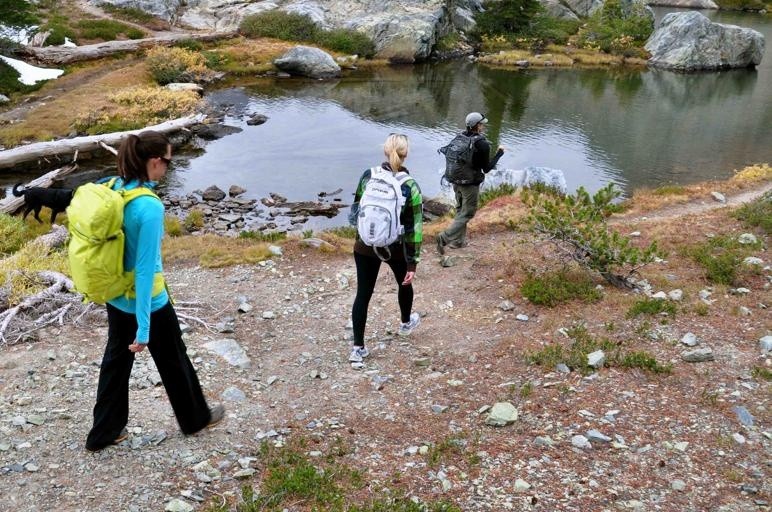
[398,312,420,336]
[435,233,445,255]
[84,427,129,453]
[448,239,468,249]
[349,345,369,362]
[205,405,225,428]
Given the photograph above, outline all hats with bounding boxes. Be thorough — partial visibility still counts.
[465,112,489,127]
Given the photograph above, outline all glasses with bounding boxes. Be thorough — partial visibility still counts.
[477,114,486,124]
[160,156,171,167]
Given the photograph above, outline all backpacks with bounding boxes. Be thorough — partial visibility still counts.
[437,130,486,186]
[350,166,414,262]
[64,175,176,306]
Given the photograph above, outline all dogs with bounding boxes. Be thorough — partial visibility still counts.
[8,176,75,224]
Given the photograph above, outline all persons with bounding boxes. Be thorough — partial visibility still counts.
[84,130,226,451]
[436,111,505,256]
[348,132,424,363]
